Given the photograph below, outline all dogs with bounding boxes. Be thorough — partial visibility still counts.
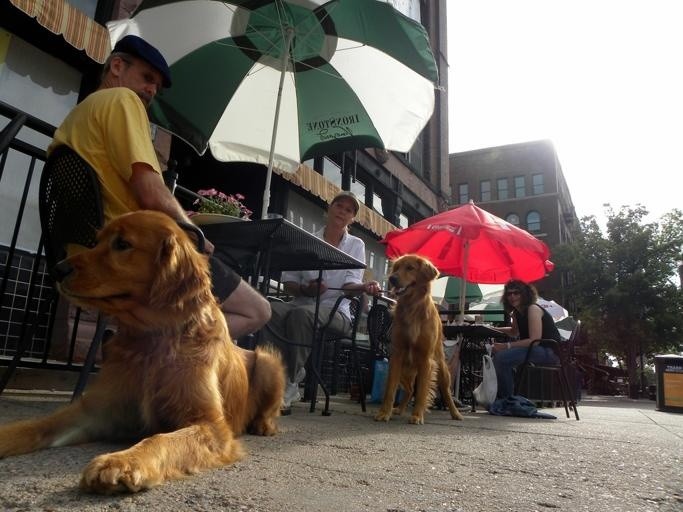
[373,253,463,426]
[0,209,287,496]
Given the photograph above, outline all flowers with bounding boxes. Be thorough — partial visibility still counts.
[186,188,252,219]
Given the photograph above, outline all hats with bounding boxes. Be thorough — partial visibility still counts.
[108,32,175,89]
[332,191,361,213]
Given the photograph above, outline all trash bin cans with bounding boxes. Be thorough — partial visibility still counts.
[655,354,683,413]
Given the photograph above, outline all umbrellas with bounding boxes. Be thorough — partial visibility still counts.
[376,199,554,400]
[105,1,440,222]
[430,276,507,310]
[465,290,570,325]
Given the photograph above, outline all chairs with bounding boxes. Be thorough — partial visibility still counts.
[368,304,446,411]
[1,145,204,405]
[515,320,582,421]
[264,295,366,413]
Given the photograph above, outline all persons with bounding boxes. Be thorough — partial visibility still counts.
[257,188,383,417]
[47,34,273,358]
[493,277,562,402]
[376,294,396,354]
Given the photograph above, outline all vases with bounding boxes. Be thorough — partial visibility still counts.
[190,214,244,224]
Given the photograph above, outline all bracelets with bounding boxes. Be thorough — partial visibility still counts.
[507,340,512,350]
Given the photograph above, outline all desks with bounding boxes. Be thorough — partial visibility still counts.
[444,326,517,412]
[198,217,367,416]
